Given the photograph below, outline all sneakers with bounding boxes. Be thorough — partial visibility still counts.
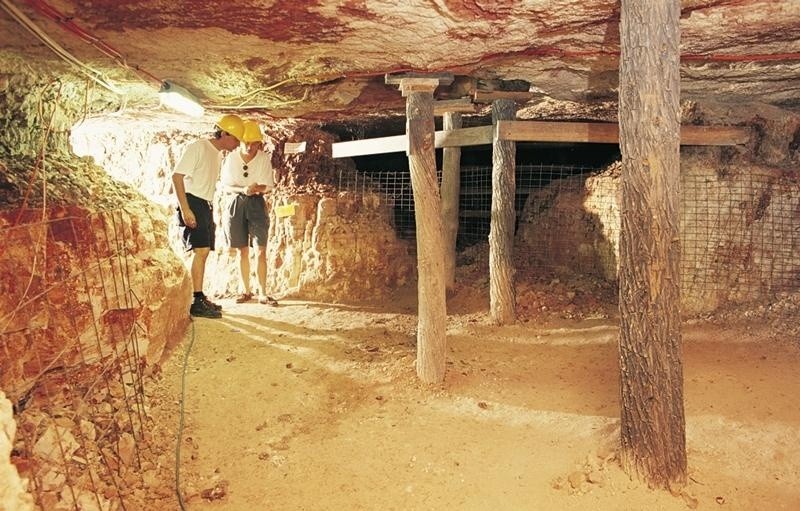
[260,296,277,305]
[237,291,251,302]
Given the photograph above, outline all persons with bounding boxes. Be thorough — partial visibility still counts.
[219,120,279,307]
[170,115,245,320]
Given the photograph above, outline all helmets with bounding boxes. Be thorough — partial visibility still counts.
[243,122,263,142]
[215,115,245,142]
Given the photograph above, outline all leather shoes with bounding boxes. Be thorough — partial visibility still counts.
[191,297,222,318]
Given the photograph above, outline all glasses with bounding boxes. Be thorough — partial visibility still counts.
[243,165,248,177]
